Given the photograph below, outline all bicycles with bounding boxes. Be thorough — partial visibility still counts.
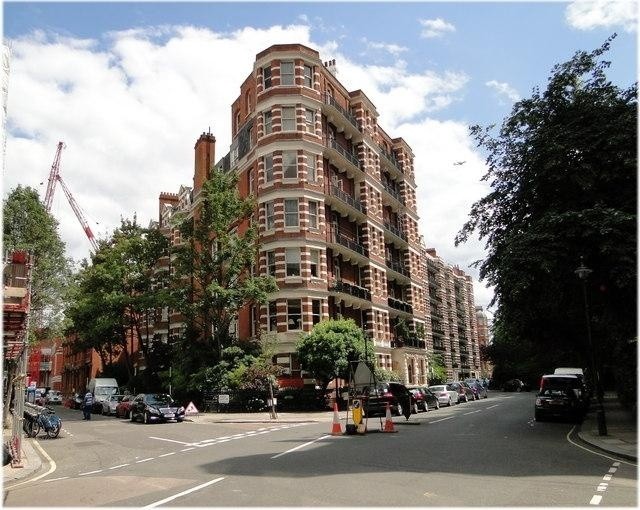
[23,402,63,438]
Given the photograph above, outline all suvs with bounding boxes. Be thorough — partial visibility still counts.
[534,367,593,425]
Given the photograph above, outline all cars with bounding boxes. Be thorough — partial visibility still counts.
[101,393,186,424]
[24,387,63,404]
[362,379,490,416]
[499,378,532,393]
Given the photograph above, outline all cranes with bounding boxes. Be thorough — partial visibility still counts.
[40,141,101,254]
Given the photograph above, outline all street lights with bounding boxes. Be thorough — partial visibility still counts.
[573,255,603,394]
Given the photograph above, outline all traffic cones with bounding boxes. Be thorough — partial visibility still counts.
[378,402,399,433]
[331,403,344,436]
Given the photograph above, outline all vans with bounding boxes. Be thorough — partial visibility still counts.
[84,378,121,409]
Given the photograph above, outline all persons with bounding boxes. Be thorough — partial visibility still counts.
[82,389,93,419]
